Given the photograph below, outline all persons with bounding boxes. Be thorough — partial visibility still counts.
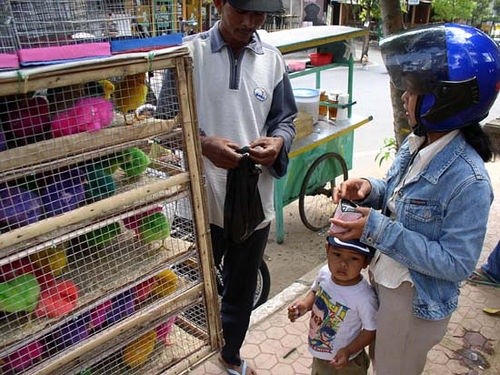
[156,0,298,375]
[328,20,500,375]
[466,240,500,288]
[288,233,379,375]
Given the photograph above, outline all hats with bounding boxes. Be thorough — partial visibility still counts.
[229,1,285,13]
[328,236,374,256]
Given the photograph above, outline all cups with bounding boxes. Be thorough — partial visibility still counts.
[330,197,364,234]
[328,99,338,120]
[318,94,328,116]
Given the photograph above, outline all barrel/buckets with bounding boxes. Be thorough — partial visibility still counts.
[292,88,320,125]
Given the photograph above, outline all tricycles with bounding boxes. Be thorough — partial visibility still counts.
[172,23,374,312]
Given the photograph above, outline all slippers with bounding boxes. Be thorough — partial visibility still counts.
[468,270,500,287]
[219,351,253,375]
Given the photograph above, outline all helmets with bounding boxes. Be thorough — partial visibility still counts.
[379,22,500,137]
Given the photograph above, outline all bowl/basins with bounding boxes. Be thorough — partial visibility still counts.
[308,52,333,66]
[287,61,306,72]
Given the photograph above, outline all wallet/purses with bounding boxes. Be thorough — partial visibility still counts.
[327,198,363,234]
